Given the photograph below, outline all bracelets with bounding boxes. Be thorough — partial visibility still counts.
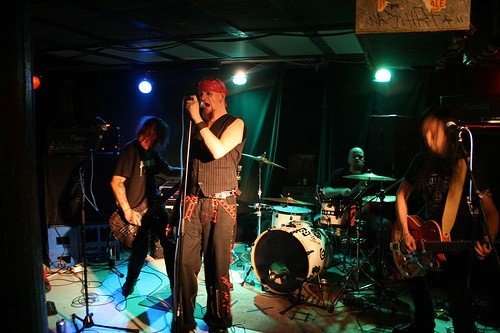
[196,120,208,132]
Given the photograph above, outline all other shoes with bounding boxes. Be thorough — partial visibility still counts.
[122,278,136,296]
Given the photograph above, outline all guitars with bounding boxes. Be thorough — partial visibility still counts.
[108,178,181,248]
[390,214,500,278]
[342,173,397,182]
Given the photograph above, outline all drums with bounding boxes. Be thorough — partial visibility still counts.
[249,219,335,296]
[269,206,312,228]
[340,217,368,246]
[318,199,352,230]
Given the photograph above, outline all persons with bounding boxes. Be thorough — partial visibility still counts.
[395,106,500,333]
[174,77,248,333]
[323,147,376,205]
[110,117,179,305]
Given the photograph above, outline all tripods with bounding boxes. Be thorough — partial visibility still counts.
[230,162,263,286]
[279,178,409,315]
[73,136,140,333]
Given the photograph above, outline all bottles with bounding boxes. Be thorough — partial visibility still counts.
[56,317,66,333]
[302,175,308,186]
[391,160,396,178]
[297,175,302,186]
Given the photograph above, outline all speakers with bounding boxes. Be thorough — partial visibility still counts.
[85,154,117,224]
[38,155,85,226]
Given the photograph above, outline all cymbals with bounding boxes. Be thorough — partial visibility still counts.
[257,195,316,207]
[362,193,396,204]
[241,152,288,171]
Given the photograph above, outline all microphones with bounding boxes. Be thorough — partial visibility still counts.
[197,102,204,107]
[445,121,460,142]
